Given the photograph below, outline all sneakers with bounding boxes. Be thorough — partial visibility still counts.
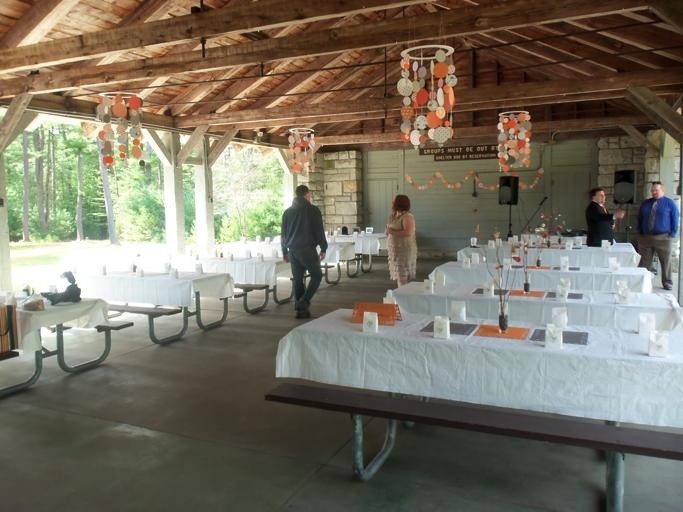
[664,285,672,290]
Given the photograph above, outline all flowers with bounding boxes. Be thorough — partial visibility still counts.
[524,209,572,244]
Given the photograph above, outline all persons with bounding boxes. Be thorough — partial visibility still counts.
[383,194,418,286]
[585,187,625,248]
[636,181,679,290]
[280,185,328,319]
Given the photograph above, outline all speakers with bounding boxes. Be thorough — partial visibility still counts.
[612,169,637,205]
[498,175,520,206]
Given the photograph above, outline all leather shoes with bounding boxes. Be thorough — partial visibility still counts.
[293,298,311,318]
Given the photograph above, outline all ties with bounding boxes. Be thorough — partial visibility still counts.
[648,200,660,229]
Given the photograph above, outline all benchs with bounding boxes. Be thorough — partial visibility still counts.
[1,348,20,362]
[60,317,134,332]
[107,303,189,317]
[263,381,682,512]
[231,231,387,291]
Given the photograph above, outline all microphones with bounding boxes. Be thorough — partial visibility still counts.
[540,196,547,206]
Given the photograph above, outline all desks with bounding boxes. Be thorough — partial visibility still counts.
[69,265,233,345]
[387,281,680,331]
[428,260,655,294]
[176,253,292,313]
[2,292,109,400]
[273,232,387,273]
[220,239,361,284]
[456,240,642,268]
[274,306,681,428]
